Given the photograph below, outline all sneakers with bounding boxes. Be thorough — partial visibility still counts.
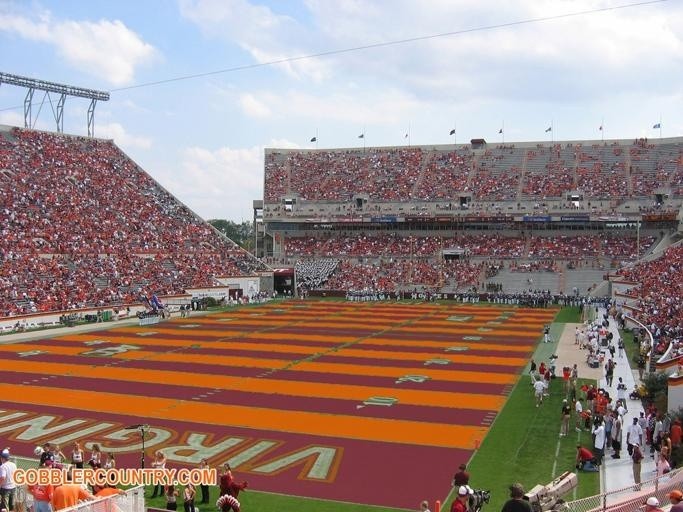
[610,454,616,457]
[613,456,619,459]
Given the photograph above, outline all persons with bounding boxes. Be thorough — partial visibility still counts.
[1,123,683,512]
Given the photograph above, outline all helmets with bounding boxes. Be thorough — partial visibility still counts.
[509,485,522,496]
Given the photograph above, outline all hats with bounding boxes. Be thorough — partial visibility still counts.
[458,485,473,496]
[0,448,9,458]
[641,497,658,507]
[631,444,638,448]
[665,490,682,499]
